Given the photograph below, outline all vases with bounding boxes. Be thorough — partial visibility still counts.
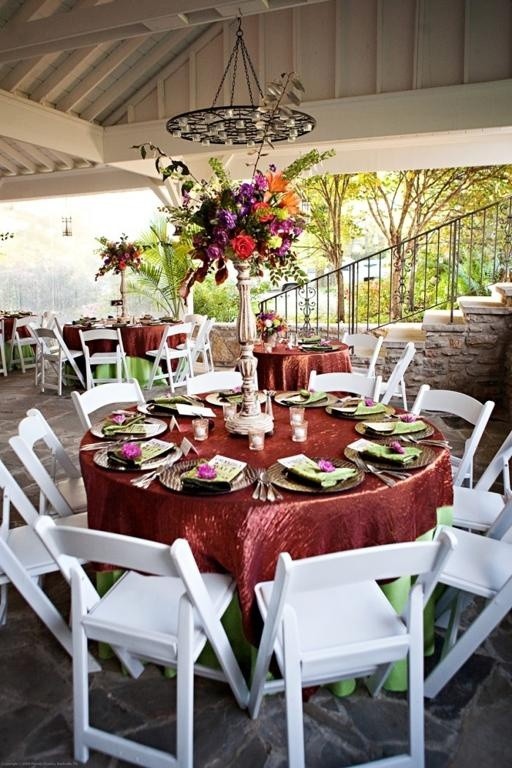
[225,262,275,437]
[120,268,130,317]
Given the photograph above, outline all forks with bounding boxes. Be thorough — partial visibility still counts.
[251,466,275,502]
[355,458,396,488]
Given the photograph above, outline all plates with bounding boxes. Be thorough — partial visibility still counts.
[205,392,268,407]
[137,400,205,417]
[266,455,365,494]
[92,440,183,472]
[298,345,340,351]
[159,457,258,495]
[354,416,435,440]
[344,439,436,470]
[274,391,339,407]
[90,417,168,440]
[325,402,396,420]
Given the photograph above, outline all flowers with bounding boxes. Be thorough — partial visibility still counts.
[129,70,337,307]
[92,233,160,282]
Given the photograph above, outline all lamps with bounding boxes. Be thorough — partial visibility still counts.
[165,16,317,148]
[61,216,72,237]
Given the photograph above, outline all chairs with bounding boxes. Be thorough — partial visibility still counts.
[144,321,197,393]
[29,324,86,397]
[413,382,495,484]
[69,377,147,431]
[176,316,216,373]
[9,406,87,518]
[6,315,40,374]
[0,310,210,354]
[424,505,512,700]
[378,341,417,410]
[34,515,249,767]
[186,370,260,394]
[308,370,383,404]
[0,461,104,674]
[451,430,512,537]
[340,332,384,370]
[247,528,457,767]
[26,323,52,386]
[79,329,130,389]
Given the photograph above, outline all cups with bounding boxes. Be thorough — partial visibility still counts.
[248,428,265,452]
[191,419,209,442]
[223,403,237,422]
[264,343,273,354]
[289,406,309,442]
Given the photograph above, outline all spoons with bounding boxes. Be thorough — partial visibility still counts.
[399,435,453,450]
[367,465,413,477]
[260,472,284,501]
